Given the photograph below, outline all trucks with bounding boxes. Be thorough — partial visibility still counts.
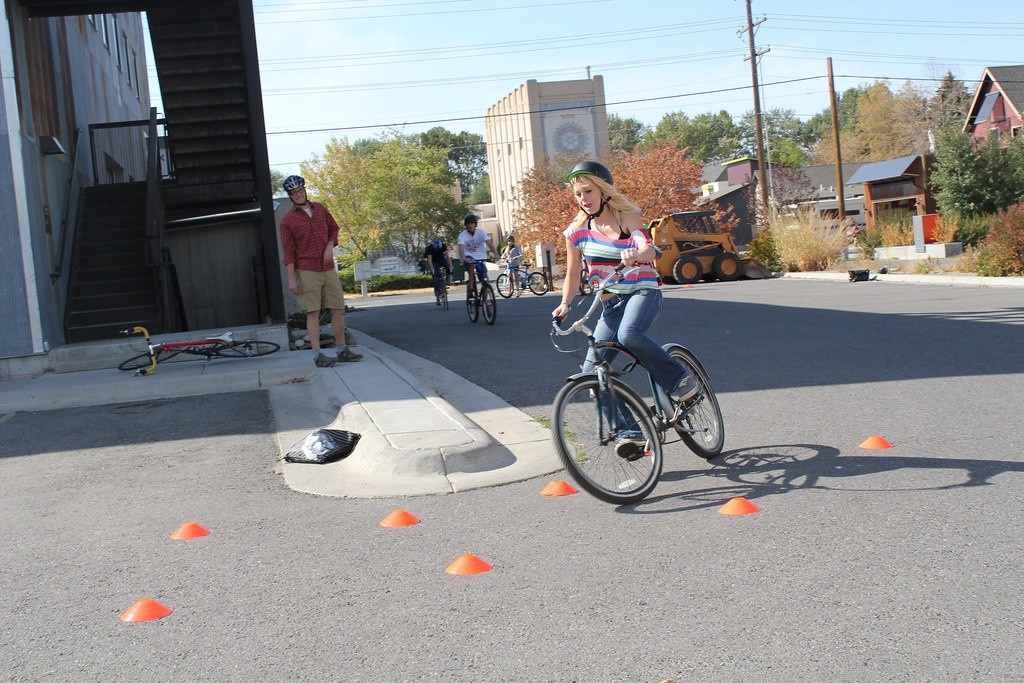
[777,197,867,237]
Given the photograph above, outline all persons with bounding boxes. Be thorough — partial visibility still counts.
[501,235,523,298]
[279,176,364,367]
[550,159,697,460]
[454,215,500,318]
[424,240,453,305]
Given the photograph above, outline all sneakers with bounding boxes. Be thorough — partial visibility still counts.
[316,353,338,367]
[677,374,699,402]
[337,347,362,362]
[614,435,650,458]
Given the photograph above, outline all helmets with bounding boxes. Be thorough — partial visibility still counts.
[567,161,613,186]
[433,240,442,249]
[465,215,477,225]
[506,235,514,241]
[283,175,305,191]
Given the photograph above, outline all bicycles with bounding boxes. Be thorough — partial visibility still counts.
[117,327,281,377]
[431,267,452,309]
[550,261,724,505]
[461,259,496,325]
[496,258,548,298]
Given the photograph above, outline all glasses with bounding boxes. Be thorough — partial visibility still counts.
[289,187,305,195]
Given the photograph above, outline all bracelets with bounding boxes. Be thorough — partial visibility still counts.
[561,300,569,306]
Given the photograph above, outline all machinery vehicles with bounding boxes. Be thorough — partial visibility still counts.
[645,210,773,285]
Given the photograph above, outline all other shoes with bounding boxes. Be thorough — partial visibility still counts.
[468,283,475,292]
[483,311,493,317]
